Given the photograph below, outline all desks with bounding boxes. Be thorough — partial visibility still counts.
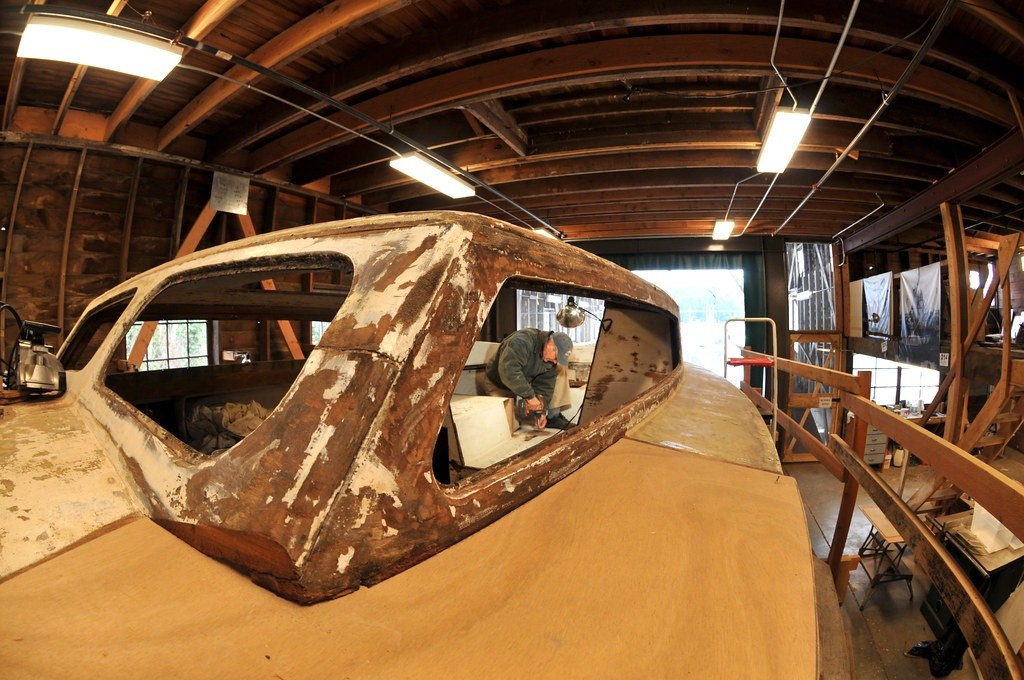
[857,504,915,612]
[894,410,946,426]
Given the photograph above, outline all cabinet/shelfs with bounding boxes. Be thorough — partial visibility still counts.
[846,414,889,465]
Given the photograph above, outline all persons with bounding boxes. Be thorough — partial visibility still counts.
[485,329,578,430]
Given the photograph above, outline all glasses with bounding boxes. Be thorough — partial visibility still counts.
[555,348,558,361]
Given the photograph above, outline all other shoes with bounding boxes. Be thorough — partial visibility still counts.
[545,413,577,430]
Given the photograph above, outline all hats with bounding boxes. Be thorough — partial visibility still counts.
[552,332,573,367]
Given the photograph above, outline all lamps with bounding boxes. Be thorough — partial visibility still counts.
[756,107,812,173]
[557,297,612,333]
[389,151,475,199]
[529,227,560,240]
[16,15,184,82]
[712,219,735,241]
